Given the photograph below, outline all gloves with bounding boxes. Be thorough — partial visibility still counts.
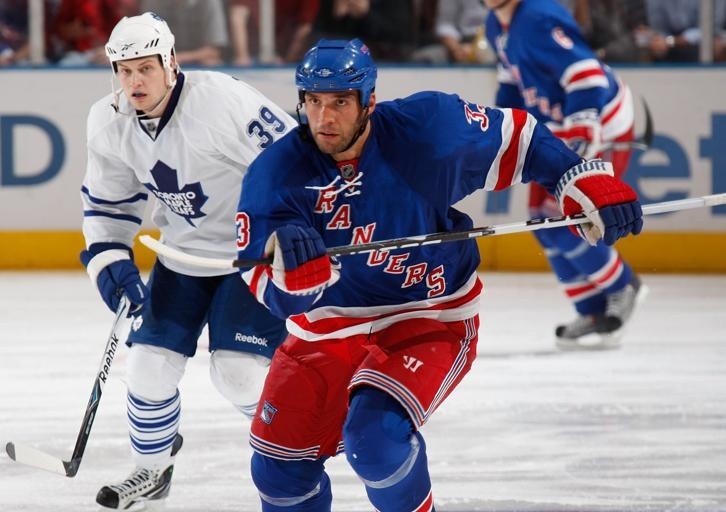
[554,159,642,245]
[81,240,151,316]
[263,226,332,316]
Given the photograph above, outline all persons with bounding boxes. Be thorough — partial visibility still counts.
[484,0,637,343]
[2,3,498,67]
[81,17,301,511]
[239,41,644,511]
[560,1,726,68]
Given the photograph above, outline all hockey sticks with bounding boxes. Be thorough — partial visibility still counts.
[138,193,726,270]
[5,290,131,477]
[580,93,653,153]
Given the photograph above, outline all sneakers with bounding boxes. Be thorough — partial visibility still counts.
[97,463,174,508]
[556,272,639,336]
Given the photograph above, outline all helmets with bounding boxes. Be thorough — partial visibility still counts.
[105,11,175,75]
[295,37,378,108]
